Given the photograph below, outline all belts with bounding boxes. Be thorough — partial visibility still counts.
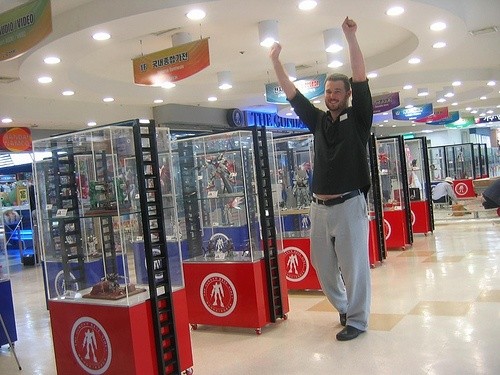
[313,189,364,206]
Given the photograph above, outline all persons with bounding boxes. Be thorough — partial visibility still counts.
[270,15,374,341]
[431,176,458,204]
[483,179,500,208]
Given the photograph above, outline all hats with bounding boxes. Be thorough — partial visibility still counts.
[444,176,453,184]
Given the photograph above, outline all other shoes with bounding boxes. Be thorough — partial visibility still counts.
[340,313,346,326]
[337,326,362,341]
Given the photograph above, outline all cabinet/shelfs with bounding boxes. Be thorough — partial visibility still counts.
[32,119,201,375]
[5,221,25,271]
[427,142,491,199]
[273,131,435,291]
[170,130,289,337]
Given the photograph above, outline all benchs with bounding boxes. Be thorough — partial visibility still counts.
[438,209,497,219]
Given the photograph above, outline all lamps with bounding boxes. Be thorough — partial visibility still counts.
[403,80,494,117]
[257,20,282,48]
[172,34,192,46]
[319,28,345,51]
[325,52,348,71]
[217,73,234,90]
